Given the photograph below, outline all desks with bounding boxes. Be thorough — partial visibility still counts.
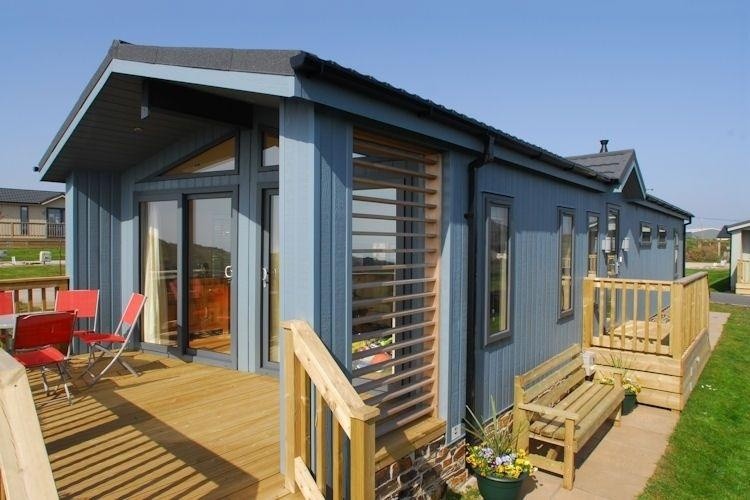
[0,307,78,335]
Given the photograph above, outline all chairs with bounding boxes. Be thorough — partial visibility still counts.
[55,286,101,368]
[0,289,19,335]
[70,289,152,388]
[9,308,80,408]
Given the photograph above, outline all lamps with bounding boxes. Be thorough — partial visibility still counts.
[601,235,616,255]
[622,237,630,251]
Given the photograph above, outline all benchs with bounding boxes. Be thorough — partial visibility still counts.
[502,340,632,495]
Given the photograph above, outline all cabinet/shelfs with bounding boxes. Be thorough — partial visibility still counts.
[160,276,234,344]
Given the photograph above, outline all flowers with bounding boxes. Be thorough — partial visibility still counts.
[594,353,645,396]
[455,395,543,481]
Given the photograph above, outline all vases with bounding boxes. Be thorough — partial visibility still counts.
[476,462,531,500]
[618,390,638,416]
